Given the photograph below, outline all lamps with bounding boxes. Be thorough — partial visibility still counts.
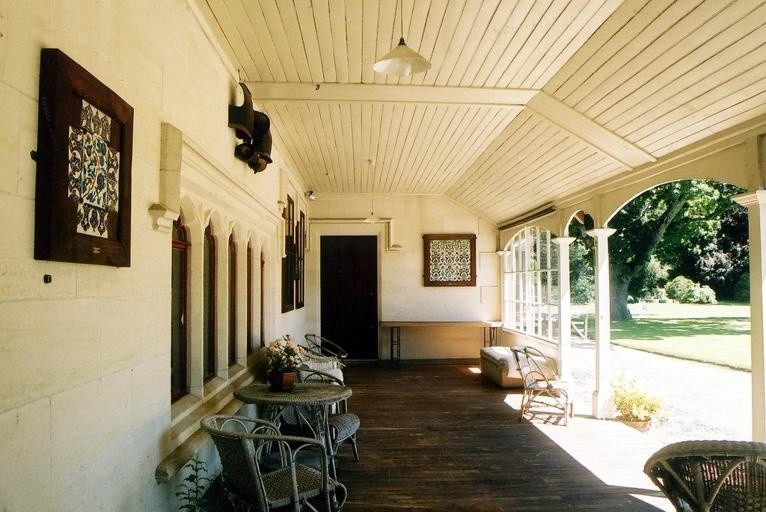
[373,0,432,77]
[363,159,382,225]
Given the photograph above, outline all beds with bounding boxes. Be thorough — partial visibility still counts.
[297,351,347,414]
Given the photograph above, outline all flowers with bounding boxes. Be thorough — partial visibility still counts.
[260,334,304,371]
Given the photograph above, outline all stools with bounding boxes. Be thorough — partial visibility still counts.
[328,414,360,462]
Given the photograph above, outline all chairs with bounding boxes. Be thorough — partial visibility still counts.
[643,440,766,512]
[524,347,574,418]
[510,345,569,427]
[200,413,349,512]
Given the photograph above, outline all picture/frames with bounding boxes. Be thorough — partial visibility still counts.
[34,48,133,268]
[421,233,476,287]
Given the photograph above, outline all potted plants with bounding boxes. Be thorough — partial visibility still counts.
[611,372,663,433]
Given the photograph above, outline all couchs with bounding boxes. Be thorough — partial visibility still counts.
[480,346,556,389]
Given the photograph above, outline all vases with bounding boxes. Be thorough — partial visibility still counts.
[270,371,297,392]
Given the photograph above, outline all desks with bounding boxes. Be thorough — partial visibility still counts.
[379,318,504,370]
[234,381,353,482]
